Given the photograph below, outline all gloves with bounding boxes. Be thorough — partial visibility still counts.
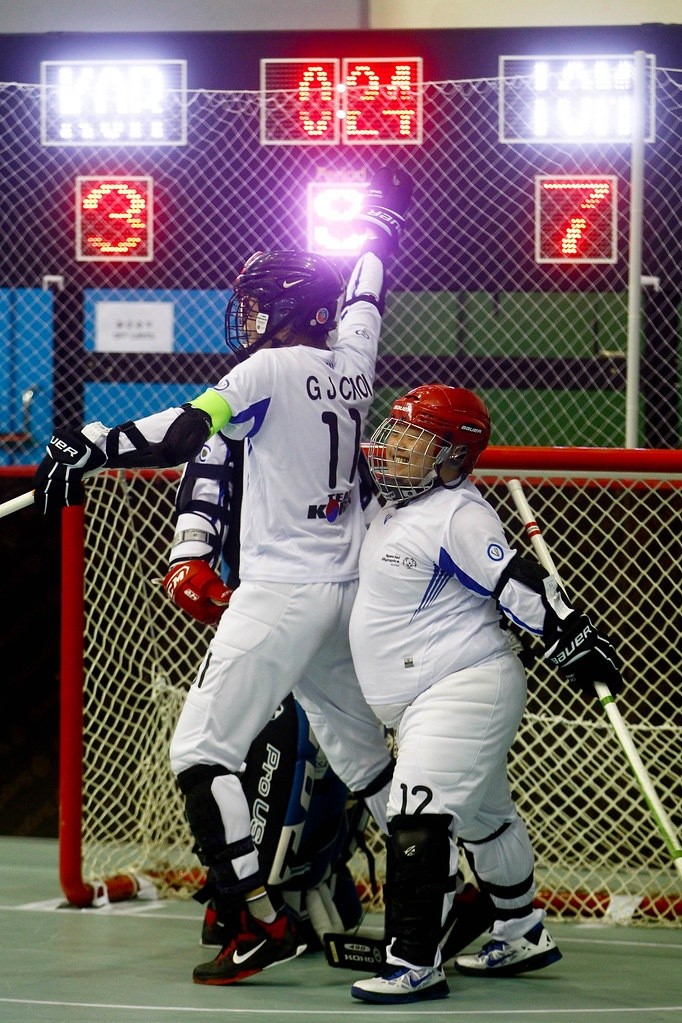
[31,427,108,522]
[543,609,624,697]
[151,558,234,625]
[359,166,414,250]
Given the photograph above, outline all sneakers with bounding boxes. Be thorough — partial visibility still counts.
[438,883,499,964]
[351,937,450,1003]
[192,902,309,984]
[201,901,229,945]
[454,907,562,977]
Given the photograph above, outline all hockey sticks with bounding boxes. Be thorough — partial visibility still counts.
[507,479,682,883]
[0,465,112,518]
[322,932,386,974]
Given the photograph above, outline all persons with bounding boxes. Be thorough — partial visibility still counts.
[348,382,623,1004]
[34,164,412,984]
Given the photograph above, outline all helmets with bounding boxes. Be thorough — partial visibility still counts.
[224,250,346,364]
[368,384,490,509]
